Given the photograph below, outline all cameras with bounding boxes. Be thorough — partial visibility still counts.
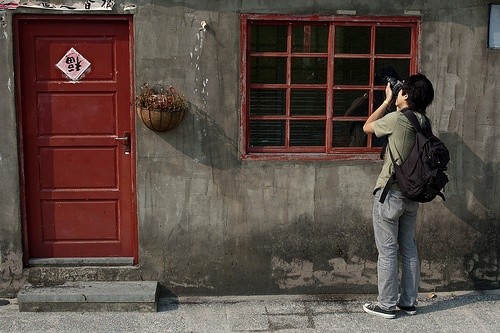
[382,76,405,97]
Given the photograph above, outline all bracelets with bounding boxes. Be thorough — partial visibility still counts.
[384,100,391,108]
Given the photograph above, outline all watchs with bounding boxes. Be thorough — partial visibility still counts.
[364,93,368,98]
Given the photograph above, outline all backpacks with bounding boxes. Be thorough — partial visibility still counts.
[387,109,450,203]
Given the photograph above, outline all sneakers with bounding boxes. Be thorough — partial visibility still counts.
[396,301,417,315]
[363,302,396,319]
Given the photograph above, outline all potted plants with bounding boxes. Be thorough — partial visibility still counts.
[134,83,187,132]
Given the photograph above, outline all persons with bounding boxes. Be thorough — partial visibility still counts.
[345,74,380,147]
[362,73,434,318]
